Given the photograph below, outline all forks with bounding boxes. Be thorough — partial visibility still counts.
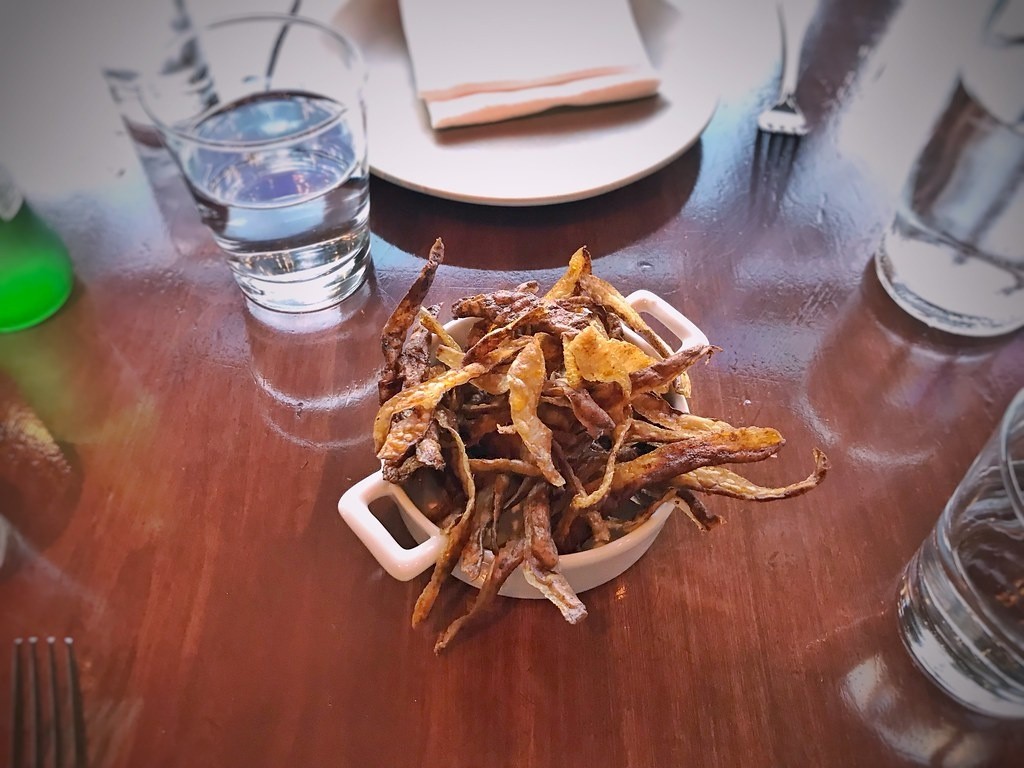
[756,0,822,138]
[8,636,86,768]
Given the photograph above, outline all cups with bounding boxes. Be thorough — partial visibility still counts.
[872,0,1024,337]
[88,1,219,138]
[892,385,1023,721]
[134,13,377,315]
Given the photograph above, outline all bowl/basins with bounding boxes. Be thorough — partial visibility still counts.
[336,287,711,601]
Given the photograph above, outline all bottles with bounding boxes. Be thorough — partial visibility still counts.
[0,160,76,335]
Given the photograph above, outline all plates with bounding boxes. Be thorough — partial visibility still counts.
[275,1,720,209]
